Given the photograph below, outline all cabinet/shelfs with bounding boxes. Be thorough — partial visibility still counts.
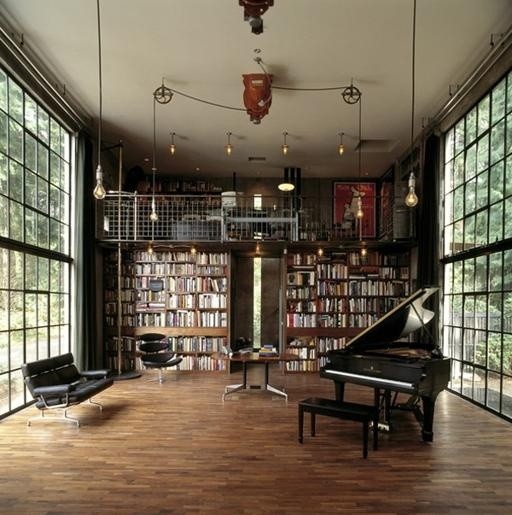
[102,247,231,375]
[283,247,414,374]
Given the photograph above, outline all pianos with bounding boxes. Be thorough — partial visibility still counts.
[319,285,452,443]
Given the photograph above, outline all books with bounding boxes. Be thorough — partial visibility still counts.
[259,344,277,357]
[285,253,412,373]
[104,252,229,370]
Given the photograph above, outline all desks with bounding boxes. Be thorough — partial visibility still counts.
[208,350,302,401]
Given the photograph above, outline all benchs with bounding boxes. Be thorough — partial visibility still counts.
[298,397,378,459]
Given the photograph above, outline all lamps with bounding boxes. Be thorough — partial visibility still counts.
[336,135,345,158]
[238,1,274,33]
[353,102,365,220]
[94,85,108,200]
[281,134,289,156]
[224,134,233,156]
[149,124,160,221]
[405,58,419,207]
[168,135,176,155]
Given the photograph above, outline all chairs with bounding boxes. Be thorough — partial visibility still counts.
[138,333,184,384]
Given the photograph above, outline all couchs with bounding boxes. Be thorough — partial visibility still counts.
[21,353,114,428]
